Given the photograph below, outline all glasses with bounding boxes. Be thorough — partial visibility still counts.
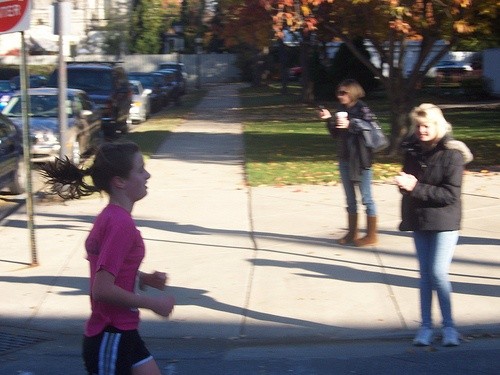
[335,90,348,96]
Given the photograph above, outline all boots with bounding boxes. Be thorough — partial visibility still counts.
[338,213,358,244]
[355,216,378,247]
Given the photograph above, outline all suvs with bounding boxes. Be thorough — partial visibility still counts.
[50,61,131,139]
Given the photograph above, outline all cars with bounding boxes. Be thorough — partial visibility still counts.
[0,112,37,200]
[3,88,103,171]
[128,61,189,116]
[128,80,153,125]
[424,60,476,89]
[0,74,48,111]
[279,66,308,83]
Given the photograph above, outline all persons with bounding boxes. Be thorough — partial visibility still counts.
[392,101,475,346]
[39,142,174,374]
[315,80,380,248]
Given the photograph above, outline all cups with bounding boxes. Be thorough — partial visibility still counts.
[335,111,348,126]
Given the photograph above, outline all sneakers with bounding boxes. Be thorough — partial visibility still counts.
[412,325,433,346]
[441,325,460,347]
